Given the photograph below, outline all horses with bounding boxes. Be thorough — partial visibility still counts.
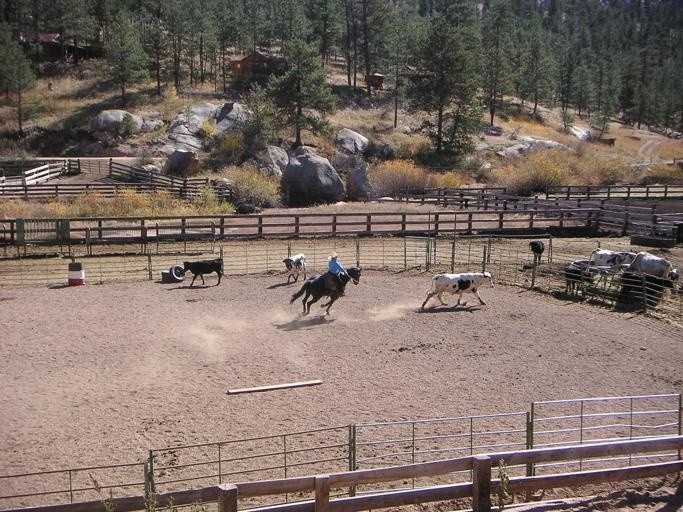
[288,266,363,316]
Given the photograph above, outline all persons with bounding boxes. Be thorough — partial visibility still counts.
[327,251,349,296]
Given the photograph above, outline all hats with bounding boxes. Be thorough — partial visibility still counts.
[328,252,338,261]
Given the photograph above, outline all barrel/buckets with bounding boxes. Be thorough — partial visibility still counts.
[68,261,85,287]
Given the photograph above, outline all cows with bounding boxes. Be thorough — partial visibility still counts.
[282,252,307,284]
[419,270,495,311]
[565,247,681,307]
[528,240,546,265]
[182,257,225,287]
